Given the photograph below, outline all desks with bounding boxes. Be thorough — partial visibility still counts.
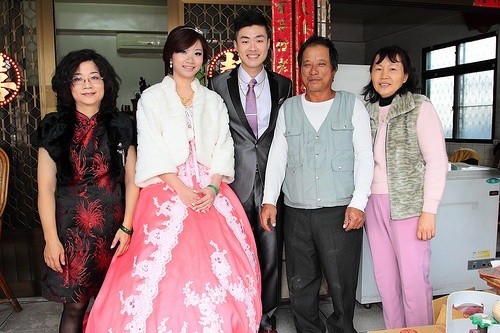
[478,263,500,296]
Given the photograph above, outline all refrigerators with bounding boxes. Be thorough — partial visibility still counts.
[355,163,500,310]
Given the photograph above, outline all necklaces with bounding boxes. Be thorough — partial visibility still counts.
[240,79,265,99]
[176,90,194,106]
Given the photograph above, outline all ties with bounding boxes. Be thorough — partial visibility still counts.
[243,79,258,139]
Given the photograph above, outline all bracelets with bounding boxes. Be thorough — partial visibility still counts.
[120,225,134,236]
[208,185,218,195]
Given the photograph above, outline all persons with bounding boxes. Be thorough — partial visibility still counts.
[85,26,262,333]
[260,35,374,333]
[354,45,448,331]
[209,12,294,333]
[37,49,142,333]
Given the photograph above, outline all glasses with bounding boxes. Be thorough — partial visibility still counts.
[71,74,104,85]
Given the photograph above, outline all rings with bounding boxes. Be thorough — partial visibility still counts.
[192,203,196,207]
[207,204,210,207]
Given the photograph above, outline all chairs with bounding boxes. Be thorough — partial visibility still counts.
[0,143,25,313]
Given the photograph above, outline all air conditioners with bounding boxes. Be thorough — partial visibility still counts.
[115,31,168,53]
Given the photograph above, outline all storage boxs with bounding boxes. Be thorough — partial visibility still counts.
[431,286,497,327]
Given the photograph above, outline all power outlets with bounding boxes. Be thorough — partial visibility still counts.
[468,257,500,271]
